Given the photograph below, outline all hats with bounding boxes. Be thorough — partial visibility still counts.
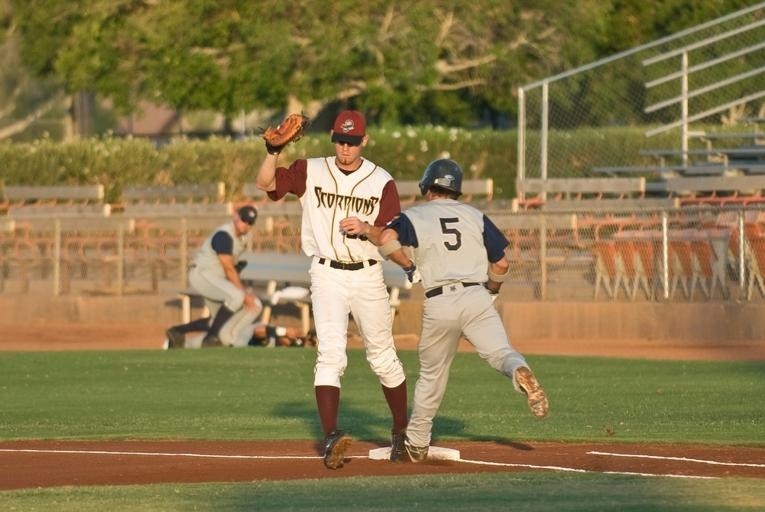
[331,110,366,145]
[239,206,258,223]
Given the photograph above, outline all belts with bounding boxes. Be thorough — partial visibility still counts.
[426,283,480,298]
[319,258,377,270]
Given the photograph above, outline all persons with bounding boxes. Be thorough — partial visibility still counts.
[165,203,264,349]
[366,156,552,461]
[253,106,412,471]
[249,322,317,348]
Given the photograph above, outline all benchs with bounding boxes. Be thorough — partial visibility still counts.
[0,175,765,353]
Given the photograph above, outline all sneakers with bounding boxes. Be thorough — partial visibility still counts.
[323,430,353,469]
[200,336,233,347]
[390,431,429,463]
[167,326,183,349]
[516,366,548,417]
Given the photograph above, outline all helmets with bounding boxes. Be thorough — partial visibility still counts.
[419,160,463,195]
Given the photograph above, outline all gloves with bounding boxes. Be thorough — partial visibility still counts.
[404,259,421,284]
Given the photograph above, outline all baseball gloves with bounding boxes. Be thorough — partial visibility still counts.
[260,110,309,154]
